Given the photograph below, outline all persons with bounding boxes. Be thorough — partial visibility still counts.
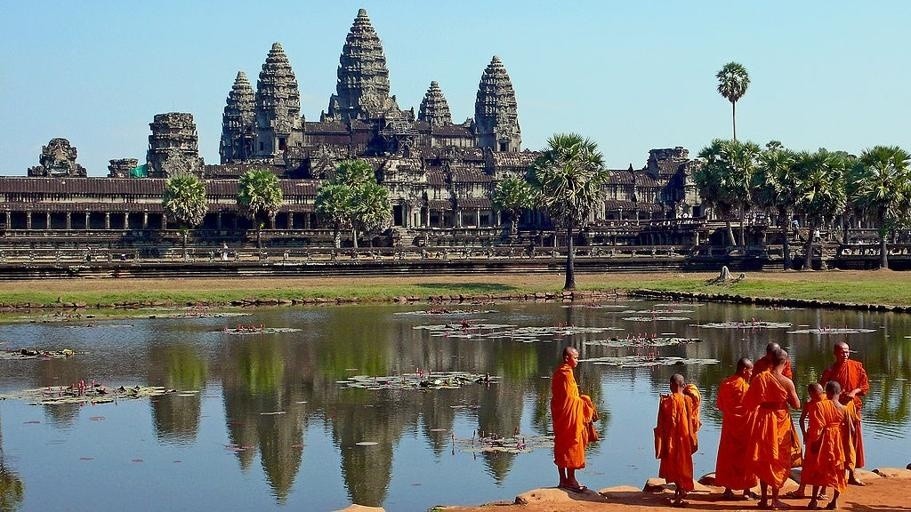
[219,243,228,260]
[814,227,821,242]
[655,342,868,510]
[550,346,600,494]
